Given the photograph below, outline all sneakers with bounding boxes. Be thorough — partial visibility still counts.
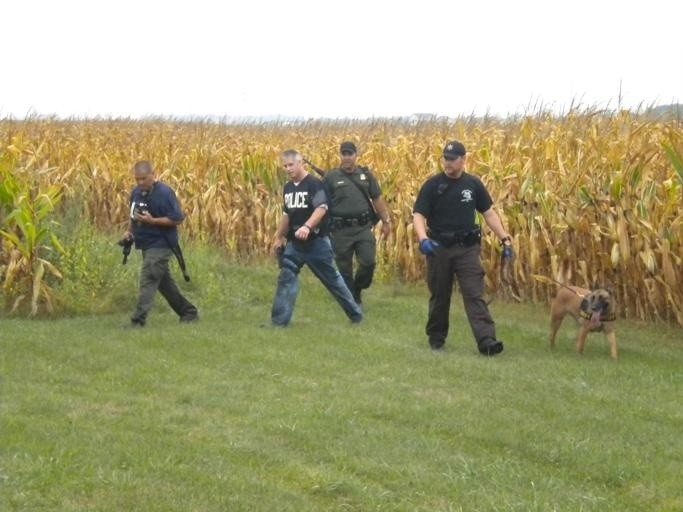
[124,321,142,329]
[179,316,199,328]
[483,341,503,355]
[259,320,285,330]
[432,344,446,353]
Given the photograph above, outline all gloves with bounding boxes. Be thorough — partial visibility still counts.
[418,239,440,258]
[501,237,513,260]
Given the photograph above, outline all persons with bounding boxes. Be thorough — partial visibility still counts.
[120,160,197,328]
[411,141,516,356]
[266,147,364,327]
[320,141,391,306]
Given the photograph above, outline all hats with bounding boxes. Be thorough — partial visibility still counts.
[340,141,357,154]
[443,141,465,159]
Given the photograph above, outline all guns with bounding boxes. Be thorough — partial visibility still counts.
[119,235,135,264]
[277,245,284,268]
[303,157,324,177]
[175,244,189,281]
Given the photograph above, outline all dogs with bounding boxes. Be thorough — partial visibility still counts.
[548,285,618,360]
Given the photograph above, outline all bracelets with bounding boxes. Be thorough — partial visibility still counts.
[302,224,311,233]
[499,235,513,246]
